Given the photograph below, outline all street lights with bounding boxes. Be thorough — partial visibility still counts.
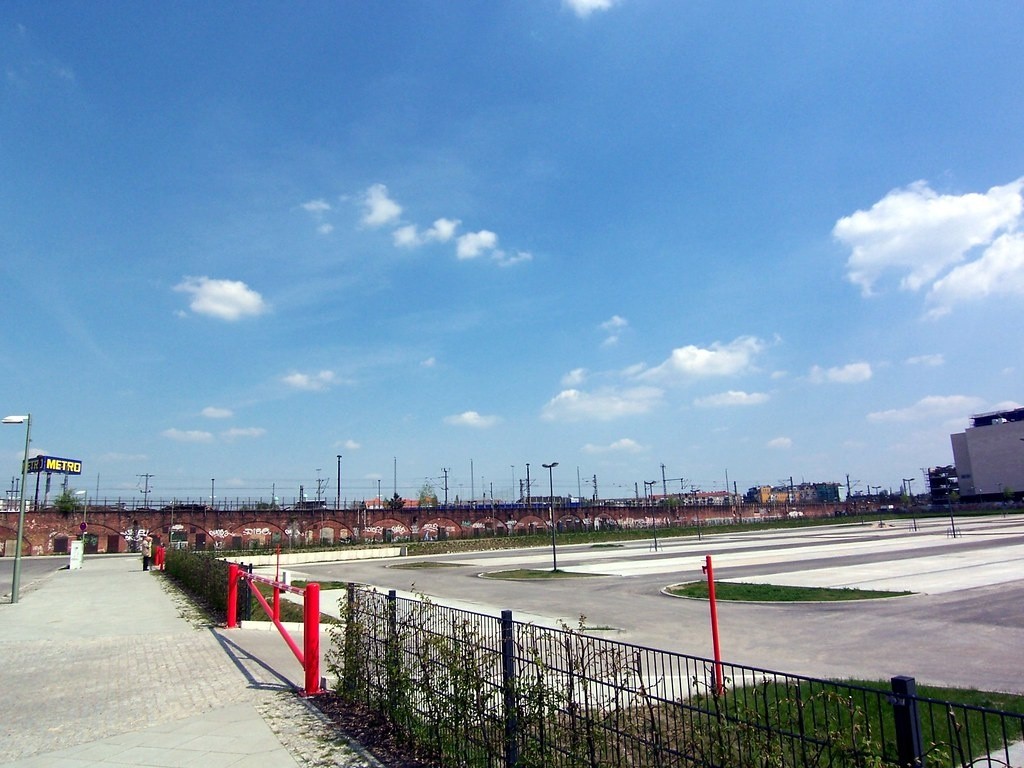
[644,480,657,551]
[903,478,917,532]
[872,485,882,529]
[1,413,32,603]
[541,462,559,573]
[691,489,701,541]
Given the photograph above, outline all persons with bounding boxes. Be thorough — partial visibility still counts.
[142,536,153,571]
